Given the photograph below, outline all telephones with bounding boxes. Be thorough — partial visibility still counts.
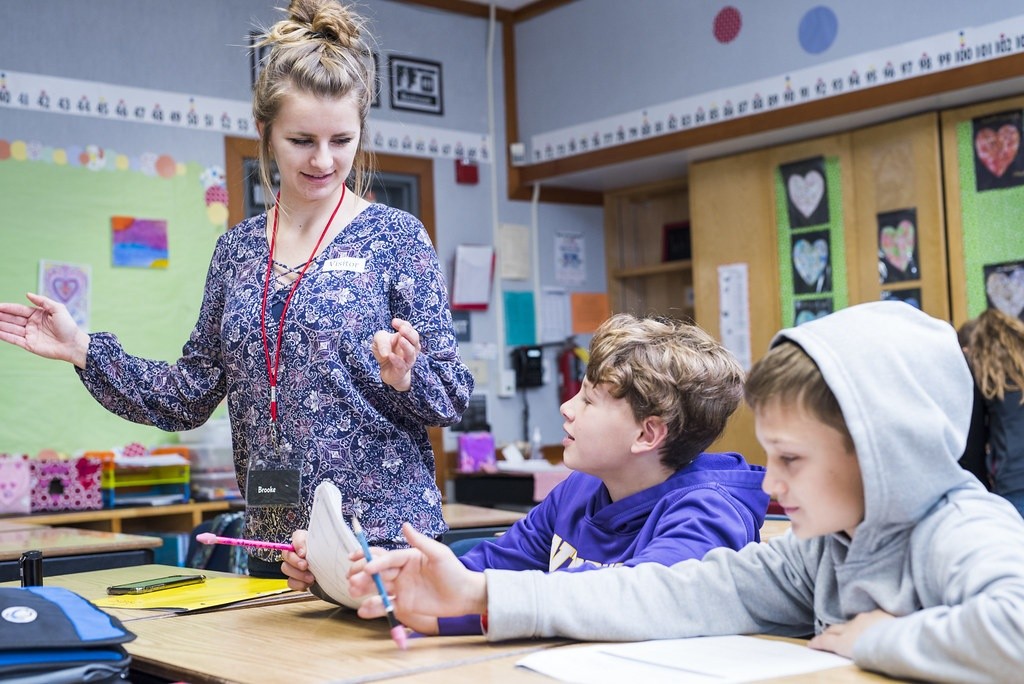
[513,344,545,390]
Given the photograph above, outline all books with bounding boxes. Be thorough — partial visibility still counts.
[88,576,318,612]
[304,482,378,610]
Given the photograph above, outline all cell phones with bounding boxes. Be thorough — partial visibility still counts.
[107,574,207,596]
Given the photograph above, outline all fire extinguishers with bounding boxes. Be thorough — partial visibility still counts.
[561,334,580,402]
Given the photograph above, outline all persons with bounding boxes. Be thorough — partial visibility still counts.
[280,299,1024,684]
[0,0,476,580]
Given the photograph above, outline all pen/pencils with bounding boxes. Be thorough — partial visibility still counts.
[195,533,295,551]
[347,514,409,654]
